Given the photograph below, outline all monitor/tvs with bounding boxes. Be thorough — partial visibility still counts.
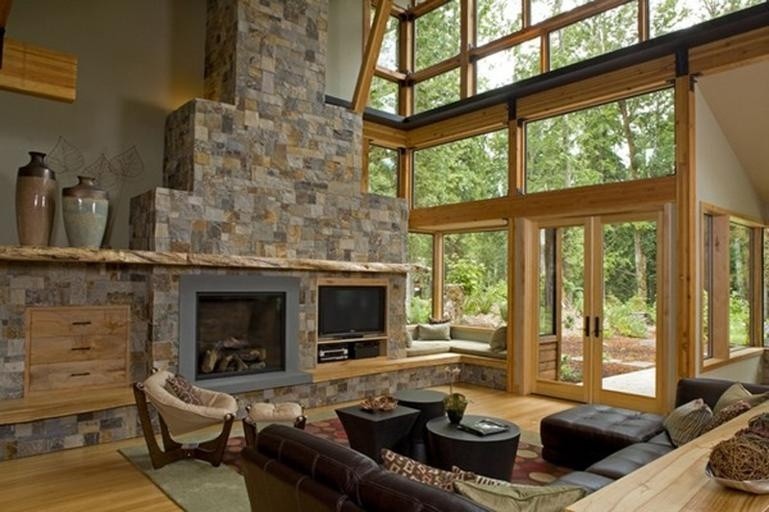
[318,285,386,339]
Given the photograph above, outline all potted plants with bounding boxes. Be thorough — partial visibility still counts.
[443,392,472,425]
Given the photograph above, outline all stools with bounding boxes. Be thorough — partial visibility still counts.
[244,401,307,448]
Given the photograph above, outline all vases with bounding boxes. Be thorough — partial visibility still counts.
[62,175,110,248]
[13,151,56,246]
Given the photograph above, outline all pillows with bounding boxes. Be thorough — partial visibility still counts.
[664,397,711,447]
[406,327,412,349]
[451,465,527,489]
[490,326,507,353]
[451,476,586,512]
[382,448,475,491]
[713,379,769,415]
[417,323,451,341]
[705,400,751,430]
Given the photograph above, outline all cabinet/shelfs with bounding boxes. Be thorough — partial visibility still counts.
[26,305,129,397]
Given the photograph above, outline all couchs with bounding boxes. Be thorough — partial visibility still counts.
[241,376,769,512]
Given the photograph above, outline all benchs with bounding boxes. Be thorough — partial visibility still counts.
[405,323,507,360]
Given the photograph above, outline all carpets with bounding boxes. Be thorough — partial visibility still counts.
[116,404,574,512]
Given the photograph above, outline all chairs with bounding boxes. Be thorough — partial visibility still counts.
[134,366,238,467]
